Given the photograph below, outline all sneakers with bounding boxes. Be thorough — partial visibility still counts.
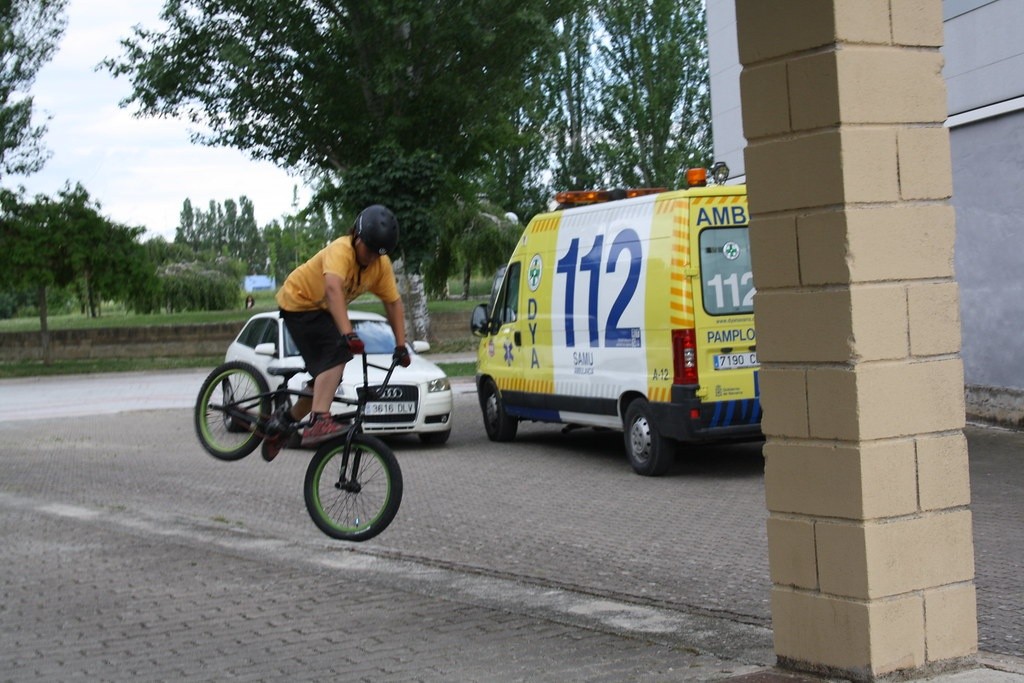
[262,412,294,462]
[300,411,352,447]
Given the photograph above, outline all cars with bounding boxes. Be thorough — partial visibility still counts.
[222,310,453,452]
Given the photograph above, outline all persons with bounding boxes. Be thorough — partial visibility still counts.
[262,205,410,462]
[245,295,256,310]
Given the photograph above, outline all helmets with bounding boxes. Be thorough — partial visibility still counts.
[350,203,401,255]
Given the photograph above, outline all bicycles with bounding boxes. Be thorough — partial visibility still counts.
[193,341,404,541]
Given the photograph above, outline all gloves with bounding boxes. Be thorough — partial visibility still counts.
[392,345,411,368]
[341,332,365,354]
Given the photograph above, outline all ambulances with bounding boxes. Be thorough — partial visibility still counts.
[469,167,763,477]
